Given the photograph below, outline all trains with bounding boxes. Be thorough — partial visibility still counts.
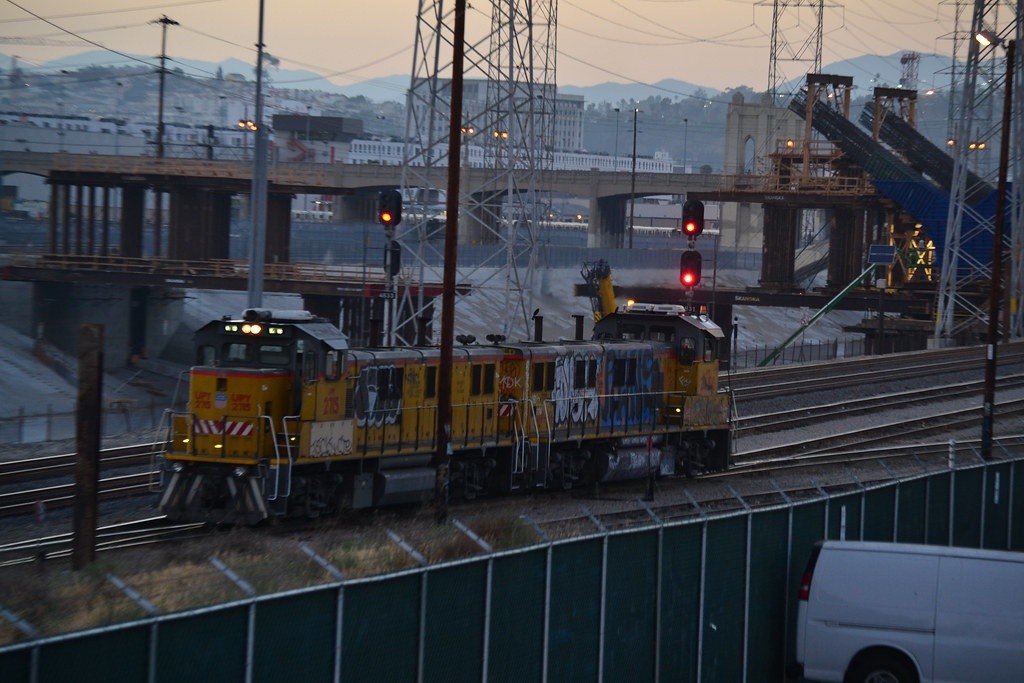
[150,256,742,536]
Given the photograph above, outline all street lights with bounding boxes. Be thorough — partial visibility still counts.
[58,69,70,153]
[304,106,314,163]
[683,118,688,174]
[974,30,1017,460]
[614,108,620,170]
[114,81,123,155]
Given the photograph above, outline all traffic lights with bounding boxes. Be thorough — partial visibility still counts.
[378,189,402,228]
[679,250,703,286]
[681,199,705,236]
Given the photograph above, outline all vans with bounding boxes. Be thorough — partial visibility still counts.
[794,539,1023,683]
